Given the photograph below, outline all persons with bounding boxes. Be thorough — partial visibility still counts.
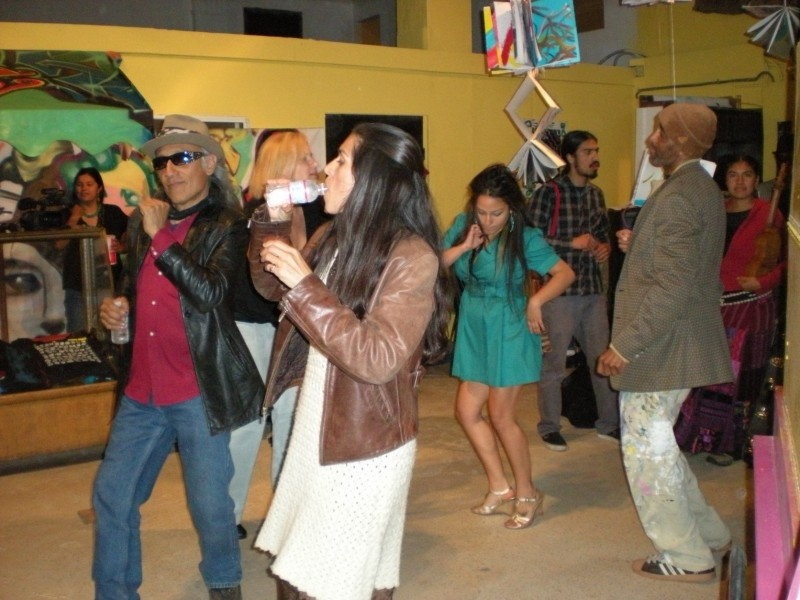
[93,116,266,600]
[228,129,327,541]
[439,161,576,530]
[675,152,788,465]
[11,166,67,222]
[597,103,735,582]
[248,124,447,600]
[525,129,623,452]
[52,168,131,332]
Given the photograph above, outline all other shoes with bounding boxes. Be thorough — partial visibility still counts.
[705,452,735,466]
[235,523,247,540]
[598,426,621,444]
[209,584,241,600]
[544,430,567,450]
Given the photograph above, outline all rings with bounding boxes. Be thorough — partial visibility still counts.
[270,266,273,272]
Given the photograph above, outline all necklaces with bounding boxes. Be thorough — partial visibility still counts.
[168,193,216,221]
[81,202,101,218]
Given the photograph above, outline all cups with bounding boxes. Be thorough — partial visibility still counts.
[104,235,116,265]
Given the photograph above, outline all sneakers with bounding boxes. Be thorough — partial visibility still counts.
[631,554,716,583]
[710,539,733,559]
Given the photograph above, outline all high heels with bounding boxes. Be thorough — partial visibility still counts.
[471,487,518,514]
[507,490,544,530]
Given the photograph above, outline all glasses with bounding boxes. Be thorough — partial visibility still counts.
[152,151,207,170]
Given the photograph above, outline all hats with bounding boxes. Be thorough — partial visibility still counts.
[659,104,717,159]
[139,114,225,163]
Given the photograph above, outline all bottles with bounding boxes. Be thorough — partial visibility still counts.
[266,180,328,207]
[111,301,129,344]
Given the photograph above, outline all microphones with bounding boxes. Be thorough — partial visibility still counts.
[17,197,40,210]
